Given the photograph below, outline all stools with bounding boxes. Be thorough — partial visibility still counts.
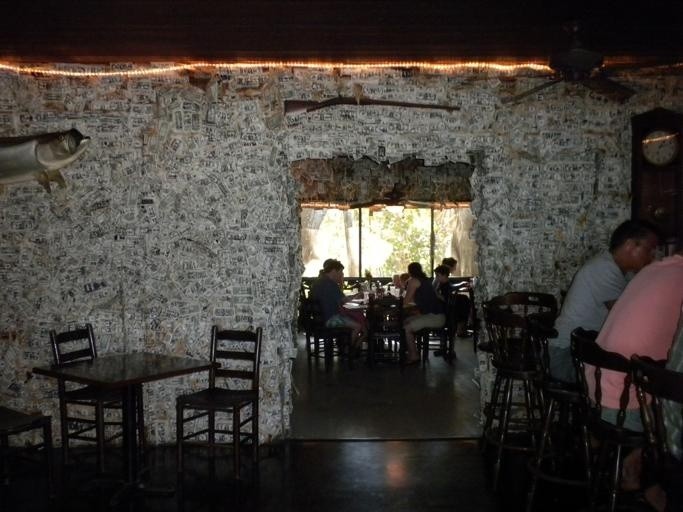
[0,405,51,479]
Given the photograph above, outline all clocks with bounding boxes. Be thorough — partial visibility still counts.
[641,129,677,166]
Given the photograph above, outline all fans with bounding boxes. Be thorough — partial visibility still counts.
[464,19,642,104]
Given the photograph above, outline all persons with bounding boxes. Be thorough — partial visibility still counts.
[644,482,669,511]
[618,448,657,494]
[568,429,608,468]
[661,294,682,460]
[584,244,682,433]
[548,217,656,387]
[310,254,474,368]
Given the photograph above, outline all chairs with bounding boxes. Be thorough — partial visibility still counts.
[628,354,681,511]
[482,309,559,478]
[298,281,475,368]
[481,291,560,429]
[569,338,631,510]
[176,325,262,480]
[517,322,596,510]
[50,324,143,474]
